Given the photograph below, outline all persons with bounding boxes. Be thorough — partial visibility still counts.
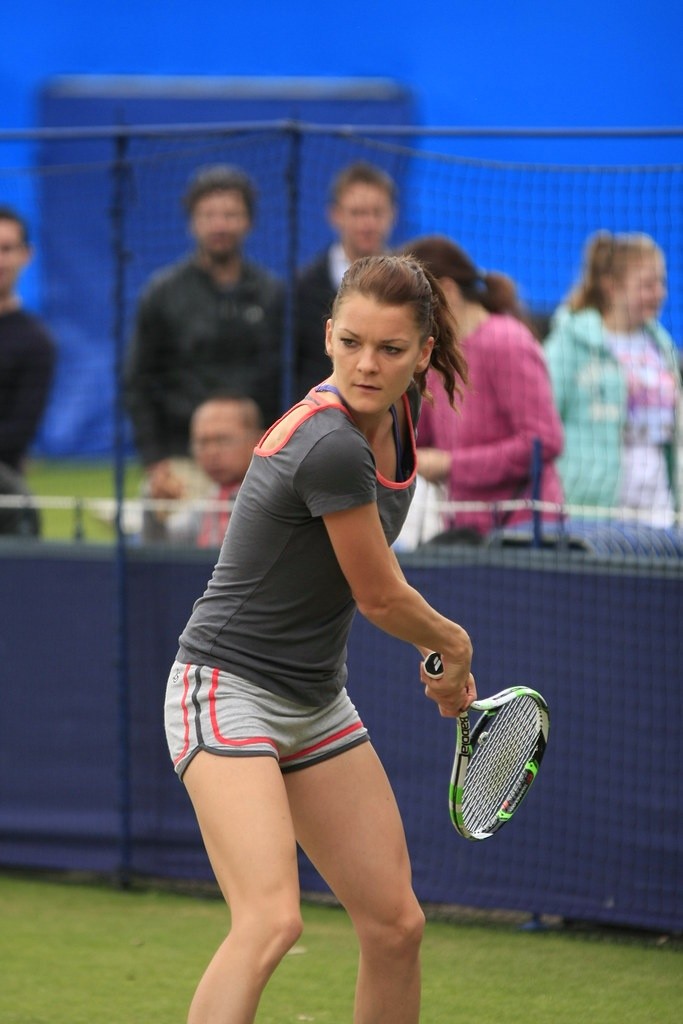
[124,164,284,469]
[542,229,683,530]
[142,394,266,546]
[292,164,399,404]
[164,257,478,1024]
[398,237,566,547]
[0,209,53,540]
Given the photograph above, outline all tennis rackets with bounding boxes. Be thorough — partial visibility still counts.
[424,652,550,843]
